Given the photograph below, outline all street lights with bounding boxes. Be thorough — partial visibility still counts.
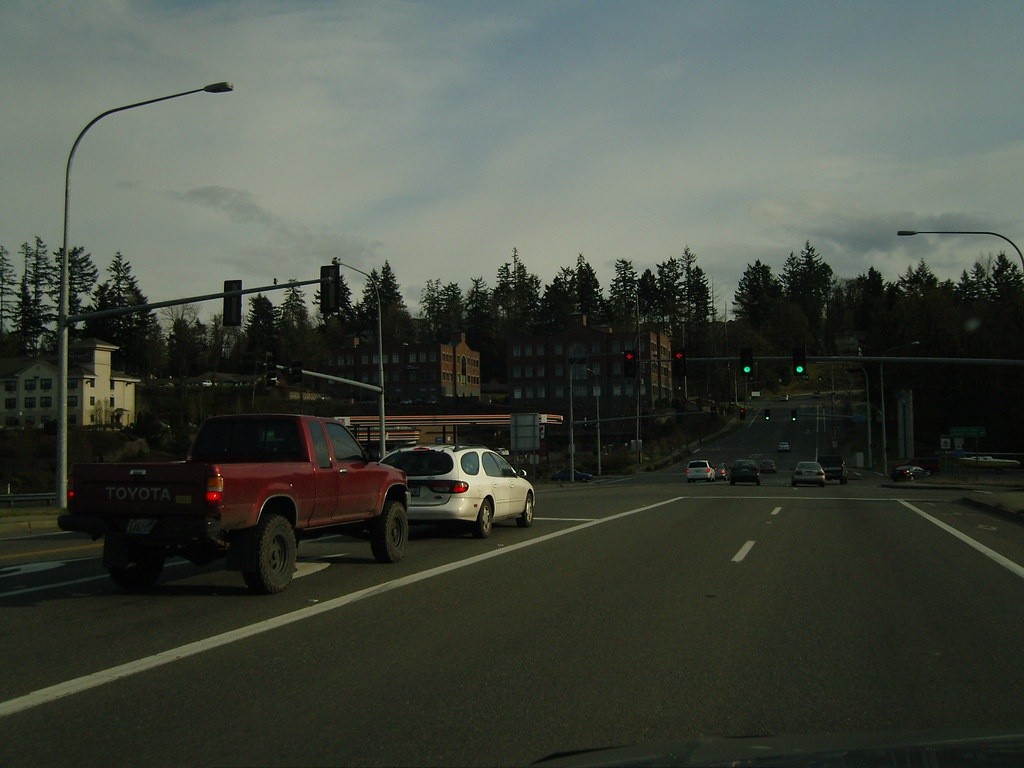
[816,361,872,468]
[587,368,601,476]
[880,341,920,477]
[898,230,1024,271]
[57,81,233,510]
[332,258,385,461]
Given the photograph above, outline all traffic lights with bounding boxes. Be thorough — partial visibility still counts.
[740,348,754,376]
[673,349,685,374]
[625,351,637,376]
[791,410,797,422]
[740,408,745,419]
[792,346,806,375]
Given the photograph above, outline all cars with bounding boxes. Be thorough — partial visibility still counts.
[685,441,825,487]
[380,443,536,538]
[765,408,770,420]
[550,467,592,483]
[782,394,788,400]
[889,461,931,481]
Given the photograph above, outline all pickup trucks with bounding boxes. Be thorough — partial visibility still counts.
[817,455,848,483]
[58,412,411,593]
